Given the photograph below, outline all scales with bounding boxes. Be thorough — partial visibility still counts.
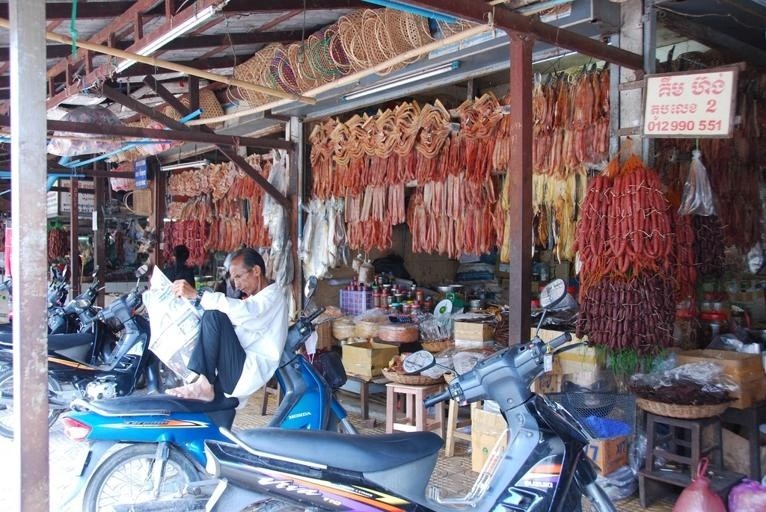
[193,275,214,307]
[434,284,465,319]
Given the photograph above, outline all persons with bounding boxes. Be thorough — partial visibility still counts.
[162,245,195,288]
[164,248,288,402]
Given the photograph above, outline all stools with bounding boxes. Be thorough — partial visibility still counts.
[384,383,445,436]
[634,413,748,512]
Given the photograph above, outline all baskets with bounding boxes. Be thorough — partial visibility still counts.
[338,289,371,315]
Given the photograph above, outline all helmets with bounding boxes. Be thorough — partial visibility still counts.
[562,369,617,416]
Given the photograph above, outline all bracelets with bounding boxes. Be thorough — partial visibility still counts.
[195,292,203,307]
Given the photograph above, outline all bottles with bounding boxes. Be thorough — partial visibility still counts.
[673,290,730,349]
[373,272,395,307]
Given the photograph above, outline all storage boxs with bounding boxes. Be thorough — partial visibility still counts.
[342,342,400,378]
[470,407,629,475]
[452,321,495,350]
[676,348,766,411]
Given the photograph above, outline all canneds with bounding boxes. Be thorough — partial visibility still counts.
[373,276,434,315]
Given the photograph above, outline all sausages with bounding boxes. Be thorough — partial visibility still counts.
[162,219,210,268]
[577,166,726,372]
[48,230,66,263]
